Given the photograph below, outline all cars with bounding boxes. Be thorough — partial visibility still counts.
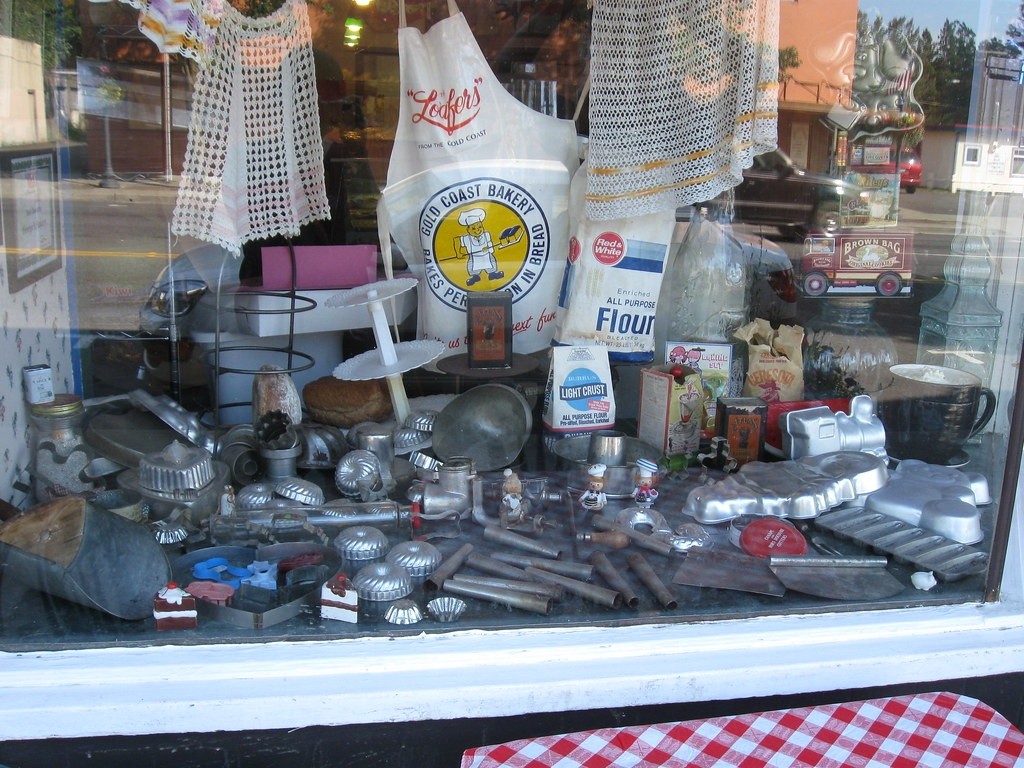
[693,142,872,246]
[137,134,800,387]
[850,143,922,193]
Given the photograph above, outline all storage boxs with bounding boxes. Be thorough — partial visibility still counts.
[191,287,418,425]
[714,397,768,474]
[468,291,513,369]
[638,363,704,458]
[664,339,748,431]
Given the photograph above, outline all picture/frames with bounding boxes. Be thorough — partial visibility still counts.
[0,142,62,294]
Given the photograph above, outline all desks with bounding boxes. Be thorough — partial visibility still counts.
[463,692,1024,768]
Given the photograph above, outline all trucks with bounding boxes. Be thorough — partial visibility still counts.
[800,226,914,296]
